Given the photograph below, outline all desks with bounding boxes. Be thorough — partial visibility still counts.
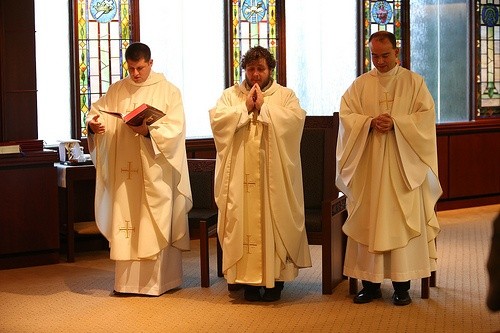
[0,147,60,271]
[52,163,105,263]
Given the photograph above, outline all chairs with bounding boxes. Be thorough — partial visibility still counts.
[186,113,437,298]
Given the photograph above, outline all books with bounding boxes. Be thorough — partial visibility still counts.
[100,103,166,126]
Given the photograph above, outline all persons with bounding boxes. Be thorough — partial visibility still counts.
[335,31,443,306]
[208,46,313,301]
[86,43,193,297]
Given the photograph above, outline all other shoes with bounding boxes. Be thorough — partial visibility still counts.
[243,287,260,301]
[262,287,282,301]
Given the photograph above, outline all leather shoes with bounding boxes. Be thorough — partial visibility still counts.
[352,286,382,304]
[392,290,412,306]
